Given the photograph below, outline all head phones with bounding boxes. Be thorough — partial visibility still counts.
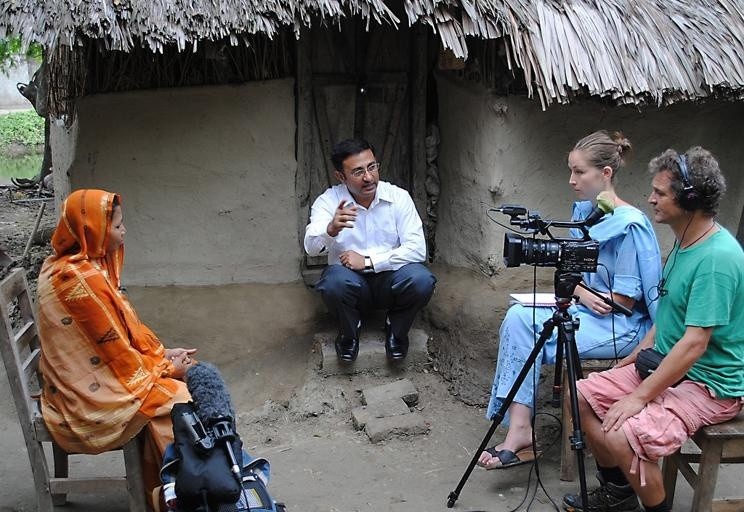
[673,154,704,212]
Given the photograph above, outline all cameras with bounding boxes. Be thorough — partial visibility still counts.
[487,204,606,273]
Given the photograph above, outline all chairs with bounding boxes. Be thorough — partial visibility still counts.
[0,267,145,512]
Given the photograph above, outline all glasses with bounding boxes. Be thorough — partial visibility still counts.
[343,162,381,178]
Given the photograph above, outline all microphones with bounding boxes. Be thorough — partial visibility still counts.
[179,362,243,485]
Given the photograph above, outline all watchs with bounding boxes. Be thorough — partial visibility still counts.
[364,255,371,269]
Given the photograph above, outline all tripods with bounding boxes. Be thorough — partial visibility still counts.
[446,272,589,512]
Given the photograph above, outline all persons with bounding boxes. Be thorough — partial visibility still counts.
[35,188,197,463]
[303,139,439,363]
[472,131,661,468]
[566,146,744,512]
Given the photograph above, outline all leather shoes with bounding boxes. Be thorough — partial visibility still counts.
[384,312,410,360]
[336,321,359,364]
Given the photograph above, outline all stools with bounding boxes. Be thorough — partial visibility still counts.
[561,359,619,482]
[662,417,744,512]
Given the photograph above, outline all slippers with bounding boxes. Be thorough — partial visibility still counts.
[482,450,544,470]
[476,445,496,467]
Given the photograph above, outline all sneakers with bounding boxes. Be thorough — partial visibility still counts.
[562,471,639,512]
